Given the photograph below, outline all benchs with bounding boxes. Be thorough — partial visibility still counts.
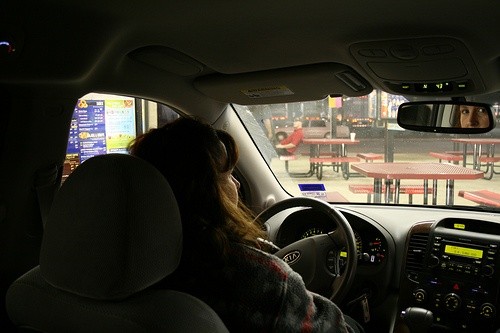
[429,152,463,164]
[457,190,500,208]
[278,152,383,180]
[349,184,434,204]
[445,151,489,165]
[480,157,500,180]
[324,192,349,203]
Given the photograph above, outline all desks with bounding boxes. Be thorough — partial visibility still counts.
[351,162,484,206]
[451,138,500,173]
[302,139,360,178]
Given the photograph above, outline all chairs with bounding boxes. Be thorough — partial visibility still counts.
[3,153,231,333]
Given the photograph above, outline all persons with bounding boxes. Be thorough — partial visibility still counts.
[268,117,303,162]
[451,105,490,128]
[127,115,410,333]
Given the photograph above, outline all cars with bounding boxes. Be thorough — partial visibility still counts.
[274,117,350,142]
